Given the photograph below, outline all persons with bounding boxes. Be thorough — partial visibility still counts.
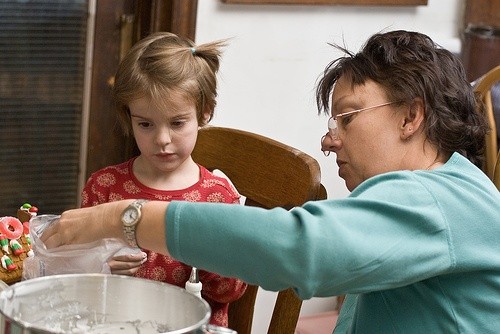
[33,30,500,334]
[80,32,247,328]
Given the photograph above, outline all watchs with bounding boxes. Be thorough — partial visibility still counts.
[120,199,149,248]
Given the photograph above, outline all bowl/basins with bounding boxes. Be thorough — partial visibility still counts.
[0,272,212,334]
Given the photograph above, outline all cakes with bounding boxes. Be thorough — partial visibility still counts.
[0,203,38,285]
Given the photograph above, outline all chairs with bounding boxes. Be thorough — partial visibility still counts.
[191,127,327,334]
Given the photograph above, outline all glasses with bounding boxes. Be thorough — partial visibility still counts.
[321,99,405,157]
